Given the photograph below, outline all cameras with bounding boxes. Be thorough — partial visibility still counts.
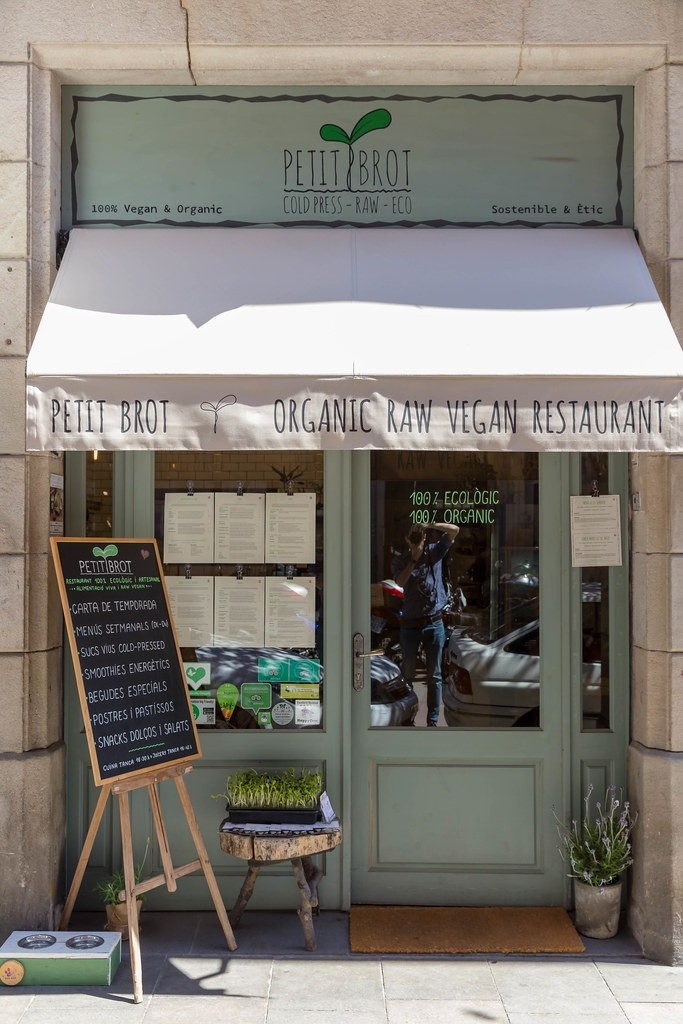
[409,532,422,544]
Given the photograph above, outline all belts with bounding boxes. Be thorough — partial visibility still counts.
[402,610,443,630]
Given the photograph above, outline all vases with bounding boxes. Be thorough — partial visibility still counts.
[573,877,622,939]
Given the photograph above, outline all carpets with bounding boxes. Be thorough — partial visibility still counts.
[349,905,586,952]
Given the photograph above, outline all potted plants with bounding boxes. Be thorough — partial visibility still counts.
[96,866,143,939]
[211,768,322,825]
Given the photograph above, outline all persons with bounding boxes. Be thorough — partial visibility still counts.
[393,514,459,729]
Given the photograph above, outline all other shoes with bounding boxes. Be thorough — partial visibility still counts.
[426,719,438,727]
[403,718,415,727]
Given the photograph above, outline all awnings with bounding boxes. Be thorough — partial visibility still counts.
[24,223,683,452]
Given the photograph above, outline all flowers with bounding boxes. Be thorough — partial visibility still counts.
[551,783,640,895]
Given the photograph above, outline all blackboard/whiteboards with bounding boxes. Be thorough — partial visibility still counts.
[56,541,199,781]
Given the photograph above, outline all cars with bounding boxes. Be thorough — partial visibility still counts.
[371,651,419,727]
[440,591,604,727]
[482,563,602,605]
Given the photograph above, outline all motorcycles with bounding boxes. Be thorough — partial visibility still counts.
[370,579,467,674]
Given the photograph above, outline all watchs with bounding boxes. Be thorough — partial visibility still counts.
[412,558,418,564]
